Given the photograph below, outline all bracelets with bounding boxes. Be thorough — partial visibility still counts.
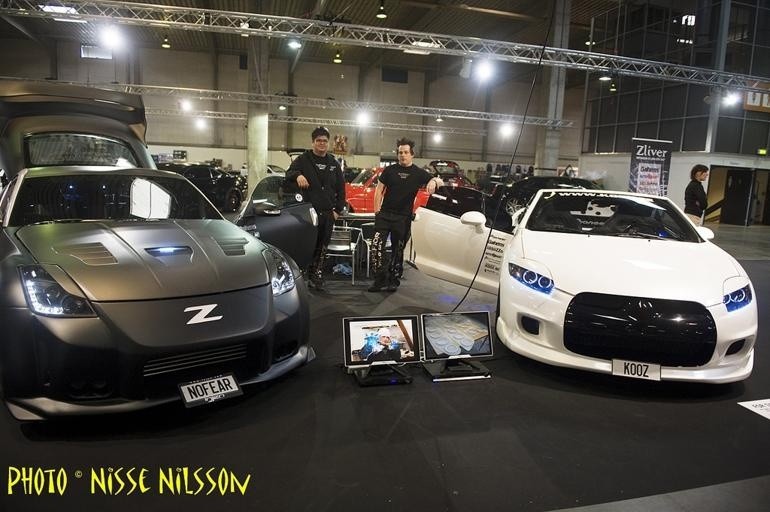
[433,179,437,189]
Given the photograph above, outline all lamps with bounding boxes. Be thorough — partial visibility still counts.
[585,31,596,46]
[333,48,342,64]
[610,82,617,93]
[161,35,171,50]
[598,71,612,82]
[376,0,387,18]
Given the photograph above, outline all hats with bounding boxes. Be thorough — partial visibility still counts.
[311,125,331,140]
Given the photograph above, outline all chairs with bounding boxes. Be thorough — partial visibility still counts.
[536,210,658,235]
[359,222,392,279]
[321,226,362,286]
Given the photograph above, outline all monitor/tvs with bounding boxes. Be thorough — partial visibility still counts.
[420,311,494,378]
[342,315,421,386]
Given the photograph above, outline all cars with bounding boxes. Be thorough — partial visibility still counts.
[0,80,316,421]
[409,184,758,384]
[152,160,287,217]
[343,160,605,234]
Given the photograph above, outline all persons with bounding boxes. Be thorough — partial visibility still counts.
[360,327,402,361]
[285,124,346,293]
[368,135,445,292]
[683,163,709,228]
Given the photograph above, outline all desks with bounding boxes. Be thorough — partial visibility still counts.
[347,211,376,275]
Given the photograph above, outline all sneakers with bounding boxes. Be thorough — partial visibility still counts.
[368,274,402,293]
